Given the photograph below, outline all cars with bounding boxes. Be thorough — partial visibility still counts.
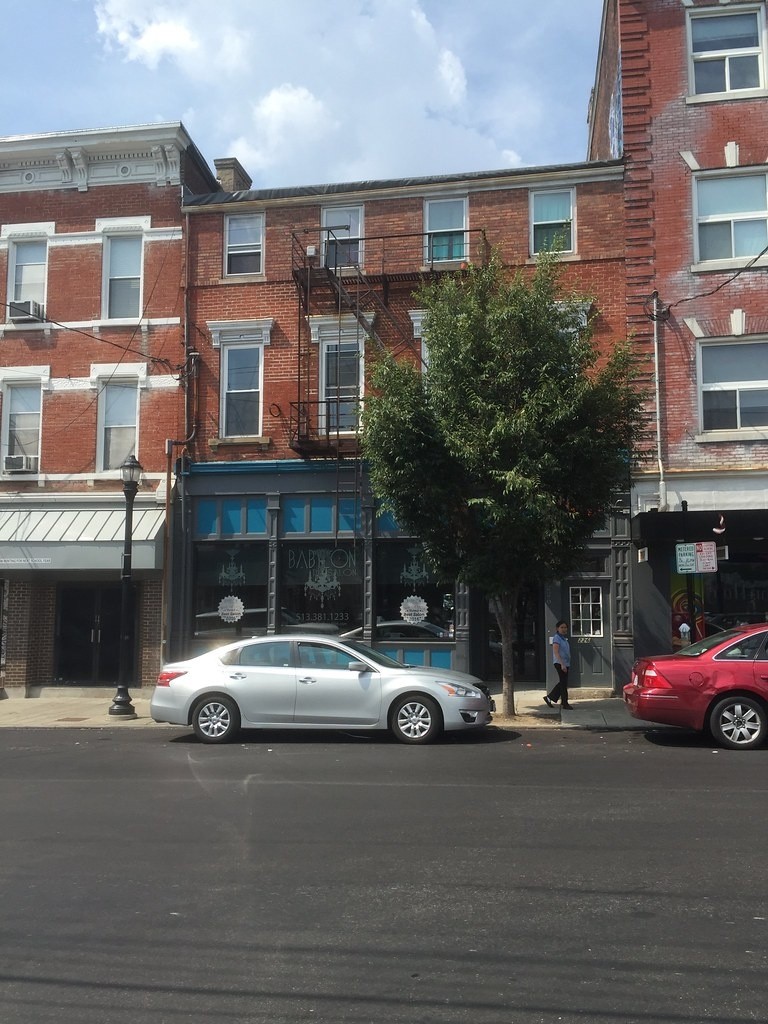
[341,621,523,681]
[621,624,768,750]
[148,631,497,743]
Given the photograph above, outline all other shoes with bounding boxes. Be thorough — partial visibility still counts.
[563,703,572,709]
[544,696,554,708]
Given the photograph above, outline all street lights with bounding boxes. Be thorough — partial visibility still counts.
[109,456,143,715]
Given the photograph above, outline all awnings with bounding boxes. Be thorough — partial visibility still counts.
[0,507,167,569]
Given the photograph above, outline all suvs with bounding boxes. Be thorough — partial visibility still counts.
[188,604,341,657]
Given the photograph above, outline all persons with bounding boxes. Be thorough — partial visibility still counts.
[543,620,574,710]
